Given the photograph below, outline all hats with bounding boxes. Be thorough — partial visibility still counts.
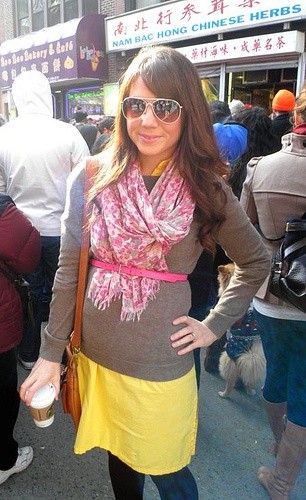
[211,122,248,162]
[76,110,88,122]
[271,89,295,111]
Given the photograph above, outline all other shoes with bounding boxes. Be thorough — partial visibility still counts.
[204,358,219,371]
[42,310,50,322]
[17,352,39,369]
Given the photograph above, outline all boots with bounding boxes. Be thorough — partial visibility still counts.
[256,419,306,500]
[262,395,289,458]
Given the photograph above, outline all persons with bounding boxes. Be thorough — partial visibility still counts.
[0,45,306,500]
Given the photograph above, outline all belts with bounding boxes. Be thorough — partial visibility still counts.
[88,258,189,282]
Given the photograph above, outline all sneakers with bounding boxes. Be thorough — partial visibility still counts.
[0,445,34,485]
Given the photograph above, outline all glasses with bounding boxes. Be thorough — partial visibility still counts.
[120,96,182,126]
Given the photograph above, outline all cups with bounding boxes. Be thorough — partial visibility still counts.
[26,383,55,428]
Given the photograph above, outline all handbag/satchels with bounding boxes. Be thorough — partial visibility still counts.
[14,275,36,333]
[268,212,306,313]
[60,331,82,434]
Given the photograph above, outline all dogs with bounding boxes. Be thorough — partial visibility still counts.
[217,264,266,398]
